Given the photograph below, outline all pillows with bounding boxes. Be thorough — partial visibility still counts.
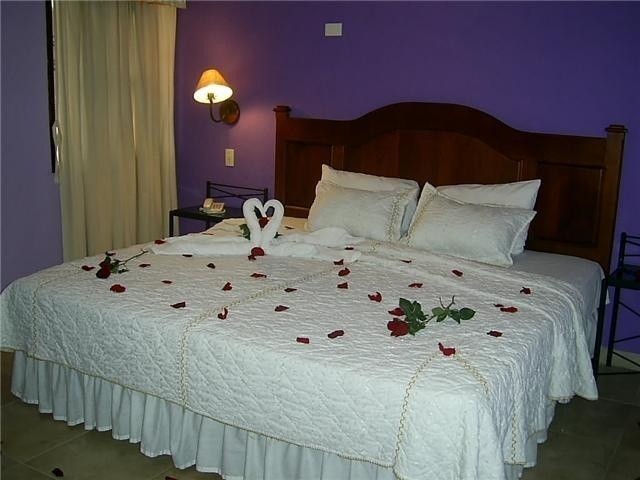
[302,162,542,271]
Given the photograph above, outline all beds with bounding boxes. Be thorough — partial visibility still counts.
[0,102,629,478]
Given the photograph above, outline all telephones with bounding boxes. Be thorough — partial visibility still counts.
[203,198,225,212]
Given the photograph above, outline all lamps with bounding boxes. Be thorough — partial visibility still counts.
[193,69,242,126]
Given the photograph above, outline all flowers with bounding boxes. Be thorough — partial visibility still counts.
[95,250,148,279]
[387,294,476,337]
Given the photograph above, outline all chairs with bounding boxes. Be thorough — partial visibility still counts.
[591,231,640,381]
[169,180,269,238]
